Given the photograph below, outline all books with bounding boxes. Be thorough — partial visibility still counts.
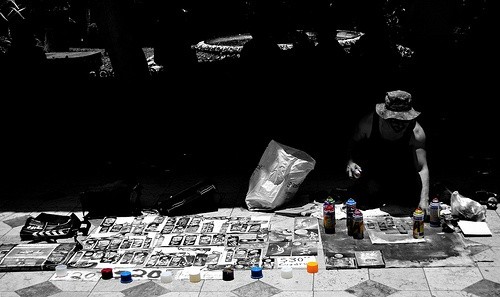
[0,243,77,271]
[326,249,385,269]
[457,221,492,237]
[69,215,320,270]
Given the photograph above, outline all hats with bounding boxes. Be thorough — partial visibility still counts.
[376,90,421,120]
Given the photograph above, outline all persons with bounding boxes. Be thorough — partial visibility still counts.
[346,90,430,220]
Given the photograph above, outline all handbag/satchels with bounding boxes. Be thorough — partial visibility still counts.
[20,212,91,252]
[80,181,126,220]
[122,176,143,216]
[156,175,219,217]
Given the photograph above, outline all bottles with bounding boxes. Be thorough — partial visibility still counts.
[324,196,335,234]
[430,198,440,227]
[346,198,364,239]
[412,208,425,239]
